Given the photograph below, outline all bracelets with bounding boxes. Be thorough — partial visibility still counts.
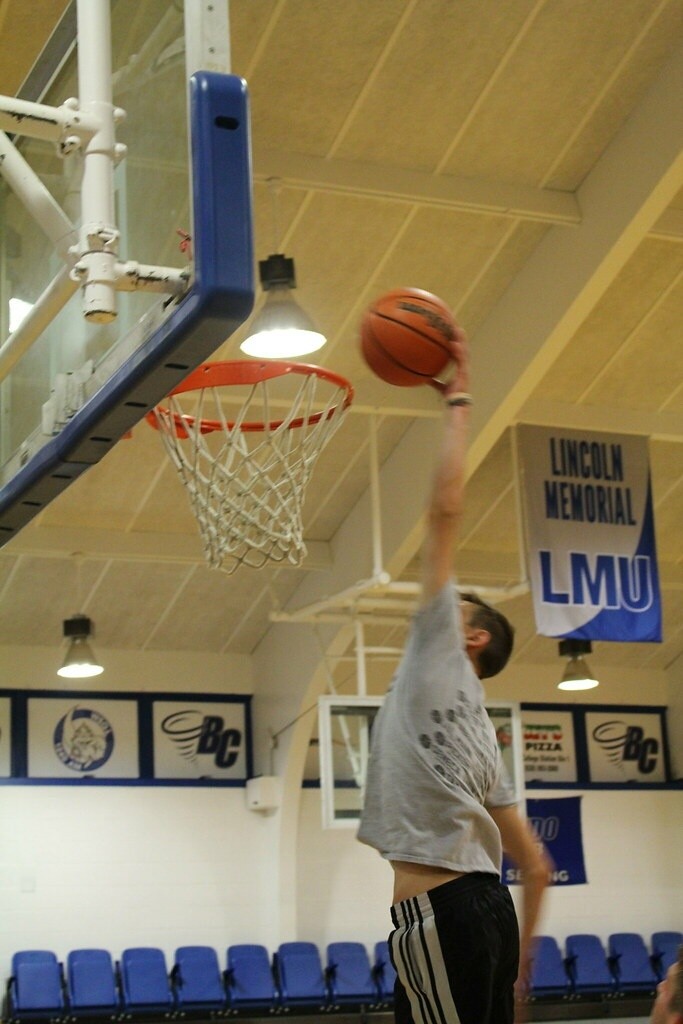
[443,391,473,408]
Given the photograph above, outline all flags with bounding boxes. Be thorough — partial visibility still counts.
[510,421,664,644]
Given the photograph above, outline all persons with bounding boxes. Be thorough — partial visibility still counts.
[353,322,555,1024]
[646,943,683,1023]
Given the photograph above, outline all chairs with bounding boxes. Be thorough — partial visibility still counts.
[175,946,225,1002]
[373,940,404,993]
[5,950,65,1009]
[223,944,277,1003]
[529,936,571,988]
[608,934,659,983]
[67,950,117,1007]
[564,935,616,987]
[271,941,328,998]
[325,942,378,995]
[115,946,171,1004]
[652,932,683,981]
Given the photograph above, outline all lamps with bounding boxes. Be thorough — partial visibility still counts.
[55,551,103,679]
[239,175,326,357]
[552,637,599,690]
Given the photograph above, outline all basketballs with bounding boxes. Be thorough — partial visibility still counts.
[359,286,457,389]
